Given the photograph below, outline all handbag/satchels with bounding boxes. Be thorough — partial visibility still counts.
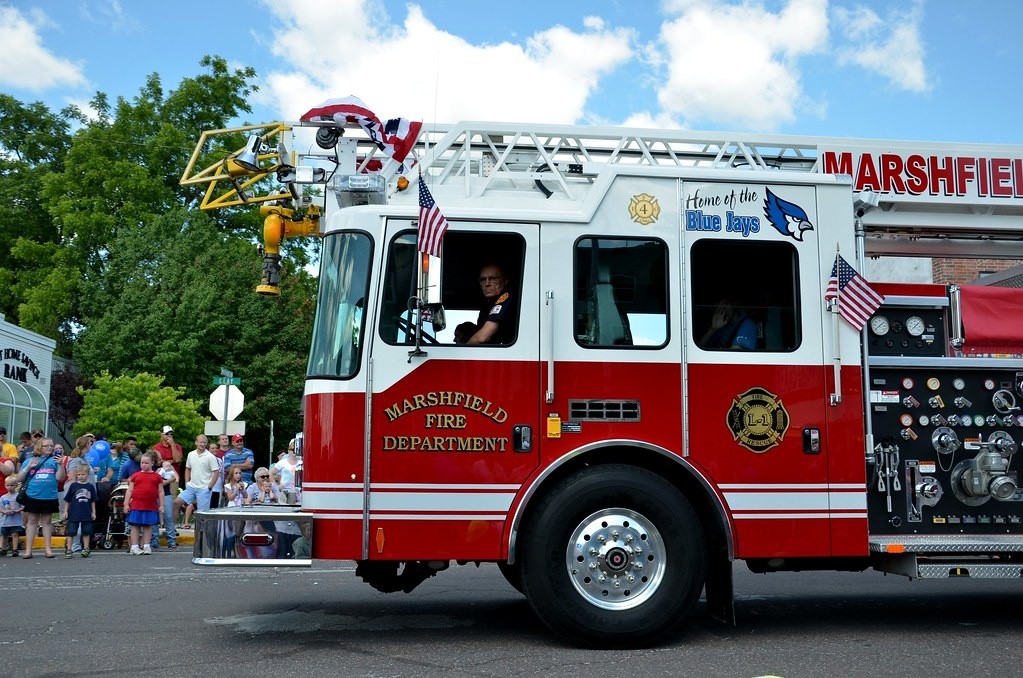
[16,491,26,504]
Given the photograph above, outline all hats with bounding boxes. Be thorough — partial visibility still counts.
[18,432,31,440]
[232,434,243,441]
[160,426,174,434]
[33,428,43,435]
[0,427,6,431]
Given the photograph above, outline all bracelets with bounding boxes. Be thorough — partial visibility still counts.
[23,469,27,473]
[208,486,212,491]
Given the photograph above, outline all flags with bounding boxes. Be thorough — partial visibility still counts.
[826,254,883,330]
[301,95,421,162]
[418,179,448,257]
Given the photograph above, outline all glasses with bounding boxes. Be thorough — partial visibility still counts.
[0,431,6,435]
[289,447,294,451]
[259,475,269,479]
[165,432,173,436]
[21,441,27,443]
[479,274,503,284]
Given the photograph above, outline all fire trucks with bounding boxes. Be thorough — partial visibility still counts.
[178,95,1022,648]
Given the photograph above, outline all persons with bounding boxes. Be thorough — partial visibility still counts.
[699,293,756,350]
[173,434,219,527]
[0,425,311,560]
[454,321,478,345]
[465,262,517,344]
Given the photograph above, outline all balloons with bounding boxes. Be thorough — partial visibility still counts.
[93,441,110,458]
[84,448,99,470]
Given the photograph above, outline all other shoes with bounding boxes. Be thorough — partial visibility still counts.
[143,545,152,555]
[12,550,18,557]
[184,523,189,529]
[81,547,90,556]
[44,551,55,558]
[130,545,144,555]
[22,553,33,559]
[64,550,72,559]
[0,550,7,556]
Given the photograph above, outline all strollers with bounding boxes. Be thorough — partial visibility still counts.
[103,480,127,550]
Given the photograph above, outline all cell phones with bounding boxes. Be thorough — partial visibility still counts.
[53,450,61,459]
